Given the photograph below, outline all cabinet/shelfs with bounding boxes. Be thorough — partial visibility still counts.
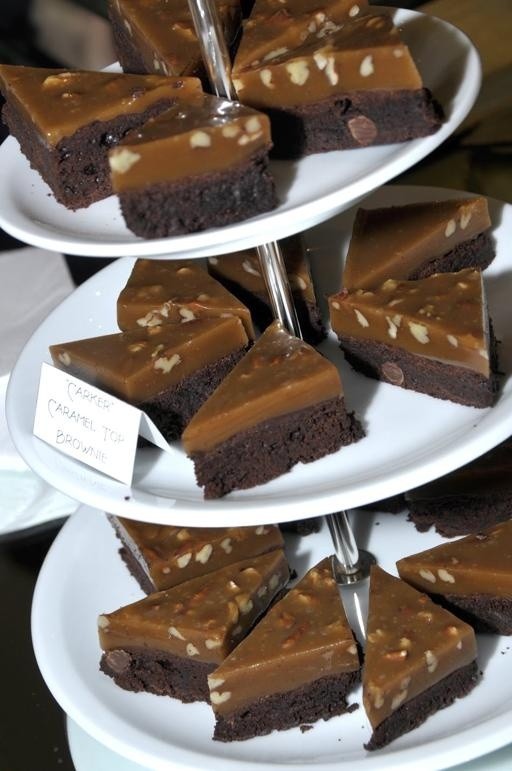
[0,0,512,771]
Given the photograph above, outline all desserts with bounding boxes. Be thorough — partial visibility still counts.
[0,1,445,241]
[49,196,494,502]
[100,444,511,752]
